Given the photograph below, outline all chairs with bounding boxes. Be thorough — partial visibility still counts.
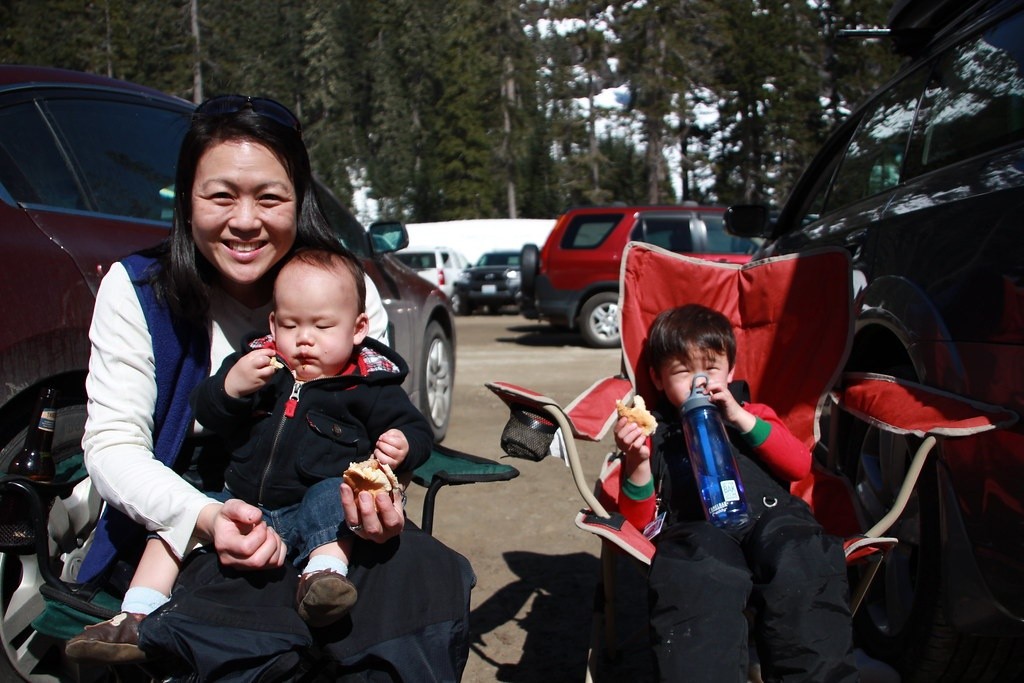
[482,234,1019,683]
[0,440,521,683]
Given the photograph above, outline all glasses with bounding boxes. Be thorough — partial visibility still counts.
[191,94,302,150]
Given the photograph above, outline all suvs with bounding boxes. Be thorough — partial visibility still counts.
[521,206,820,349]
[394,251,522,315]
[722,2,1024,683]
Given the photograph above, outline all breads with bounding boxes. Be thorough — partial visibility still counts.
[343,459,398,512]
[615,394,658,436]
[269,358,284,368]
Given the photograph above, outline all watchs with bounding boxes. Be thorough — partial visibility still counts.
[398,487,407,506]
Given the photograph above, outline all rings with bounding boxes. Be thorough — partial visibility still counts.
[347,524,364,533]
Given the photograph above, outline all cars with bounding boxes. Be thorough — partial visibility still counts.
[0,65,456,473]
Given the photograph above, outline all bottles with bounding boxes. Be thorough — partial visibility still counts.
[678,373,749,529]
[6,385,60,539]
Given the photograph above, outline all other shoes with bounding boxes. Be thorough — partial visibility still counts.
[295,568,359,629]
[64,611,146,662]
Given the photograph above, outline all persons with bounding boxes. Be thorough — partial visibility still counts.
[64,238,434,663]
[614,303,853,683]
[77,94,476,683]
